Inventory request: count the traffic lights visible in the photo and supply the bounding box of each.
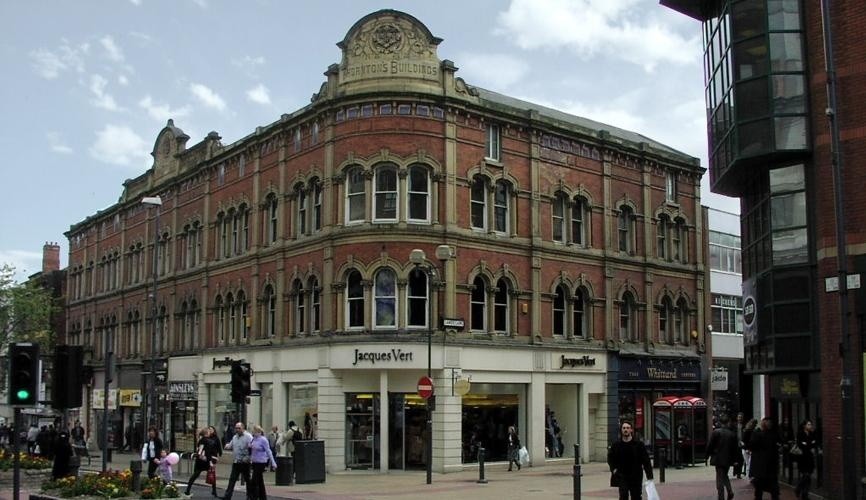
[9,342,39,407]
[230,362,252,400]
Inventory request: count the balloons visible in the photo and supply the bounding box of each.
[166,452,179,465]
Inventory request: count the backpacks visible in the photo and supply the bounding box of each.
[292,428,302,444]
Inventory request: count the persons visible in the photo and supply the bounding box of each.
[607,421,655,500]
[121,420,136,452]
[143,425,163,479]
[222,422,277,499]
[185,426,222,499]
[505,425,522,472]
[267,420,303,472]
[700,411,823,500]
[155,448,172,483]
[1,417,87,480]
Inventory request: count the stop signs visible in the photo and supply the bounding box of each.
[417,376,433,399]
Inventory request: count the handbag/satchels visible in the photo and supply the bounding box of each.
[519,447,529,464]
[643,480,659,500]
[207,467,215,484]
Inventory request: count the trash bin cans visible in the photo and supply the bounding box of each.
[275,456,295,486]
[107,448,118,462]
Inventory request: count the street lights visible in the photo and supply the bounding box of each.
[138,194,162,428]
[408,244,453,486]
